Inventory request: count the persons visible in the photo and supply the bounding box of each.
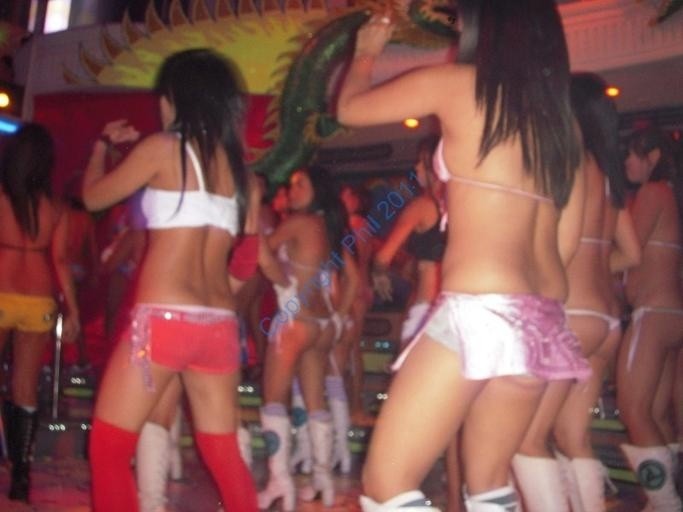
[508,71,642,510]
[0,123,682,510]
[79,49,266,510]
[332,1,584,511]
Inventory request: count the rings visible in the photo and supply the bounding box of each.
[379,16,389,24]
[367,18,375,24]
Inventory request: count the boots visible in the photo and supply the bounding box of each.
[8,403,39,498]
[253,376,351,512]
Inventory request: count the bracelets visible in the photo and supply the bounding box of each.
[92,132,110,147]
